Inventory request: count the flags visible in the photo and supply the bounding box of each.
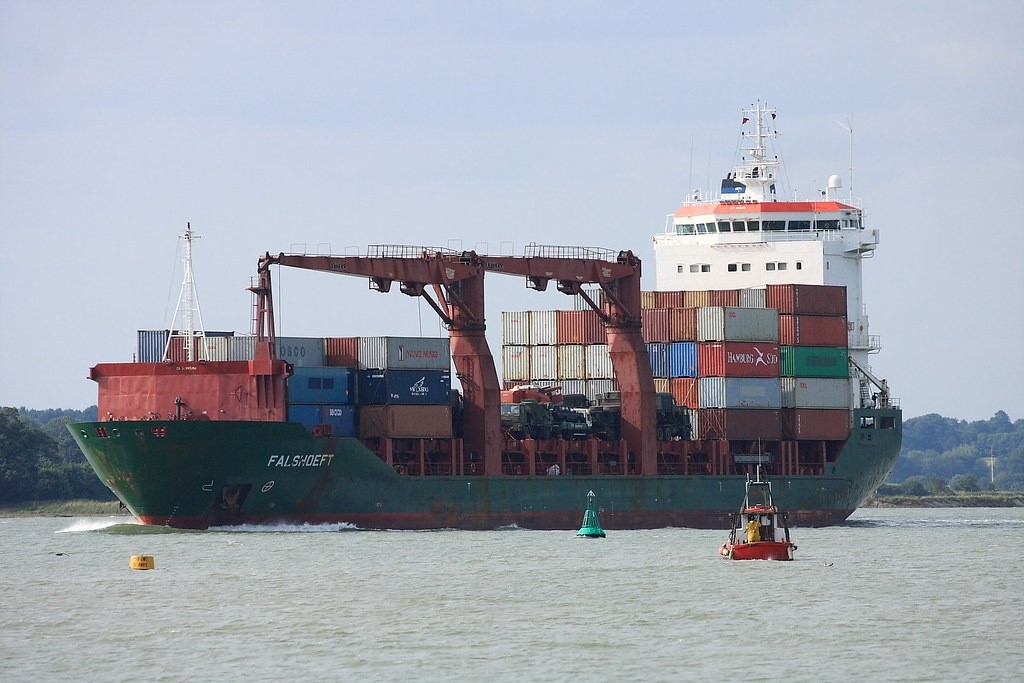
[743,118,749,125]
[772,114,776,120]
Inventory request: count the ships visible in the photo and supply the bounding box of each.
[65,103,904,539]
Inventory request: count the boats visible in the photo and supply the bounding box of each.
[719,467,799,563]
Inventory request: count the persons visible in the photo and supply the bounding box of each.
[872,392,878,409]
[705,459,713,475]
[745,516,762,544]
